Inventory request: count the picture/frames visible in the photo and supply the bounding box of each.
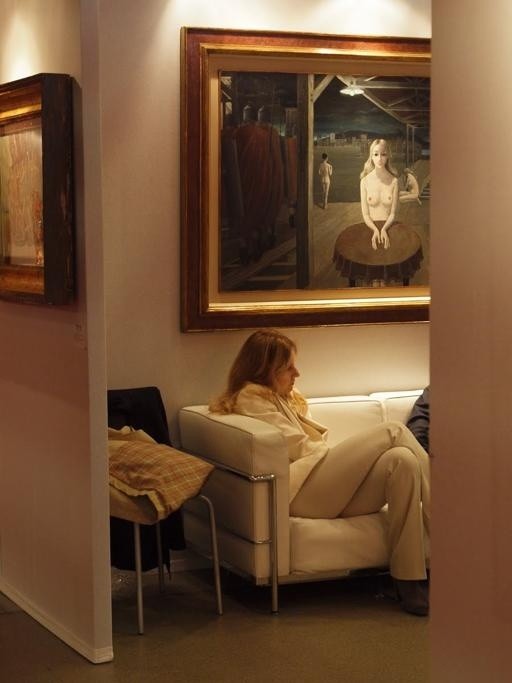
[1,74,74,307]
[181,28,430,334]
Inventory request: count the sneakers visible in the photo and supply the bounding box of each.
[392,578,429,617]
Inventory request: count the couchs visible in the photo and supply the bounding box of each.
[177,389,427,614]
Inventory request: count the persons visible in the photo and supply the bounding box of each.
[398,167,423,206]
[359,137,401,251]
[317,152,333,210]
[405,380,431,455]
[207,327,431,620]
